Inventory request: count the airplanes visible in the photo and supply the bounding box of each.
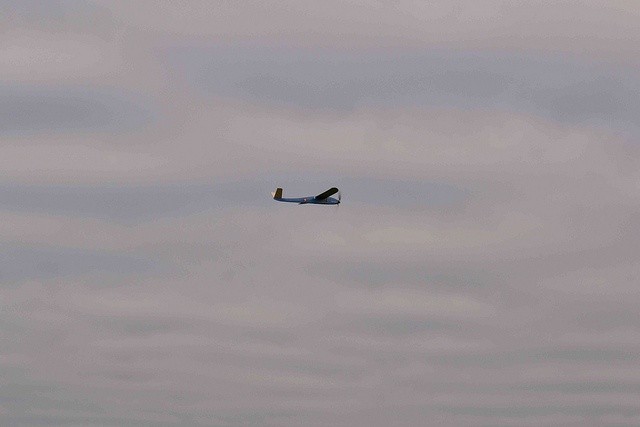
[270,186,343,205]
